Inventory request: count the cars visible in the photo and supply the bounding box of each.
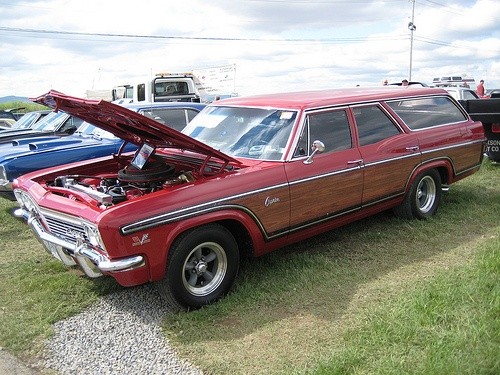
[11,85,487,312]
[427,87,480,102]
[0,103,207,202]
[0,106,85,144]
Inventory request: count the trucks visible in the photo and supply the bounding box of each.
[457,89,500,162]
[132,70,221,103]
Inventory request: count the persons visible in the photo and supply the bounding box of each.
[475,79,490,99]
[382,80,388,87]
[401,79,410,87]
